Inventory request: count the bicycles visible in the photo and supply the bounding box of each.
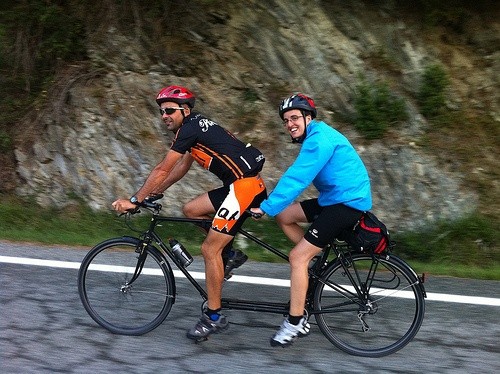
[77,194,428,359]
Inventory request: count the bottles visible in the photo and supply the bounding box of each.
[168,236,193,266]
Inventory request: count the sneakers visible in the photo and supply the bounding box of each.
[309,254,329,275]
[224,249,248,281]
[270,316,311,349]
[185,312,230,343]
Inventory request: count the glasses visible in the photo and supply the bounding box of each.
[159,107,184,115]
[280,114,304,126]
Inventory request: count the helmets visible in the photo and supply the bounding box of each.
[279,94,317,118]
[156,85,196,108]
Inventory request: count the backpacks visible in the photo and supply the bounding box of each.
[345,211,391,255]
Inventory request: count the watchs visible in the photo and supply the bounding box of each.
[130,196,140,206]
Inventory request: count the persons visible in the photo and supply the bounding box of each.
[112,86,268,344]
[250,93,372,347]
